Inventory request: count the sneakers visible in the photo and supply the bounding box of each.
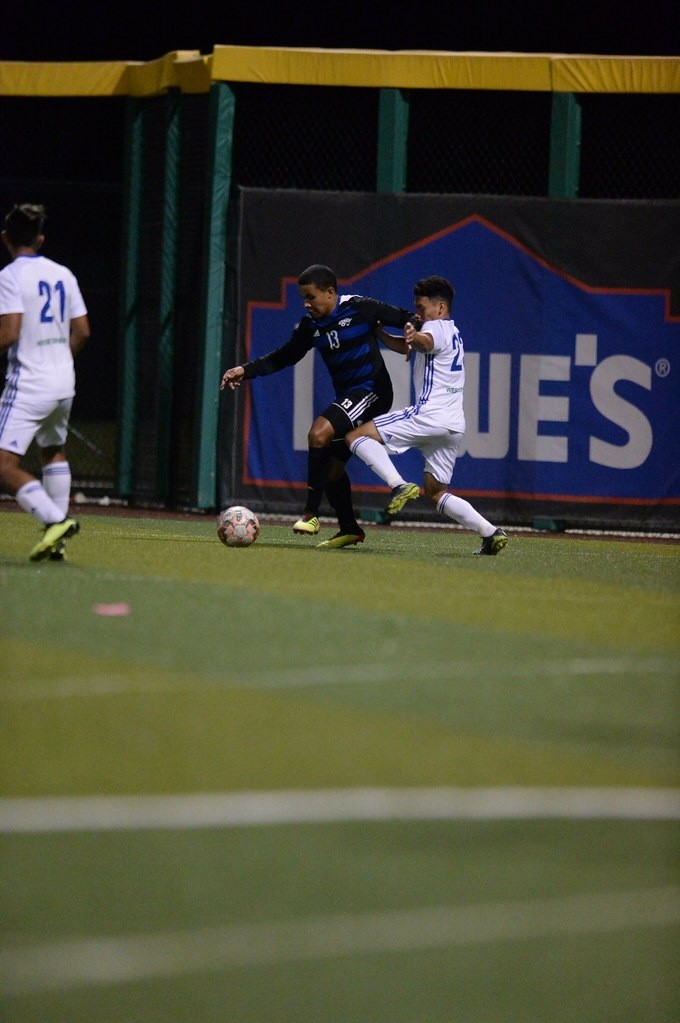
[385,482,420,515]
[28,516,80,561]
[472,527,508,556]
[292,513,320,535]
[50,544,66,561]
[316,525,365,548]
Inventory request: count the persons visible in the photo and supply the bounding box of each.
[219,264,426,550]
[0,202,90,562]
[344,275,510,557]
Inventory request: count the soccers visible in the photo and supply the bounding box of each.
[216,505,261,548]
